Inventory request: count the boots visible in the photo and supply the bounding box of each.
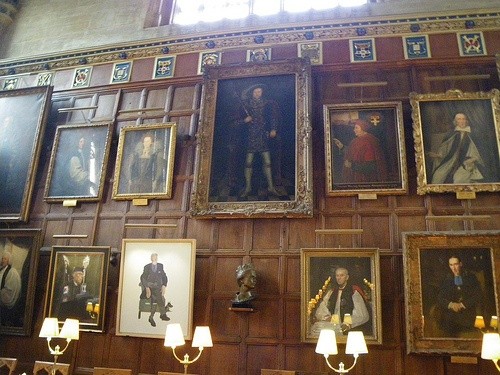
[237,165,252,198]
[262,166,280,194]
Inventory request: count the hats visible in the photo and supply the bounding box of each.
[244,83,267,97]
[354,119,369,131]
[74,267,84,272]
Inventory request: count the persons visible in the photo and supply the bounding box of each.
[229,262,257,307]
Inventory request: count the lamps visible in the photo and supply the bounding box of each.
[163,323,213,375]
[474,315,499,334]
[480,333,500,372]
[330,314,353,333]
[38,318,80,375]
[315,329,369,375]
[85,302,100,320]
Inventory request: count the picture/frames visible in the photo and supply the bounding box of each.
[401,34,432,59]
[0,228,43,337]
[348,38,377,63]
[42,121,115,203]
[115,239,198,341]
[1,77,18,90]
[408,88,500,196]
[456,31,487,57]
[71,66,94,88]
[401,230,500,355]
[246,48,272,62]
[0,84,54,224]
[185,63,316,219]
[196,51,222,75]
[41,245,112,336]
[297,42,324,66]
[322,101,410,198]
[109,61,133,84]
[151,55,177,78]
[111,121,178,200]
[298,246,384,346]
[35,71,56,86]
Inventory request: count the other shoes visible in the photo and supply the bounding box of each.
[149,319,156,327]
[160,315,170,321]
